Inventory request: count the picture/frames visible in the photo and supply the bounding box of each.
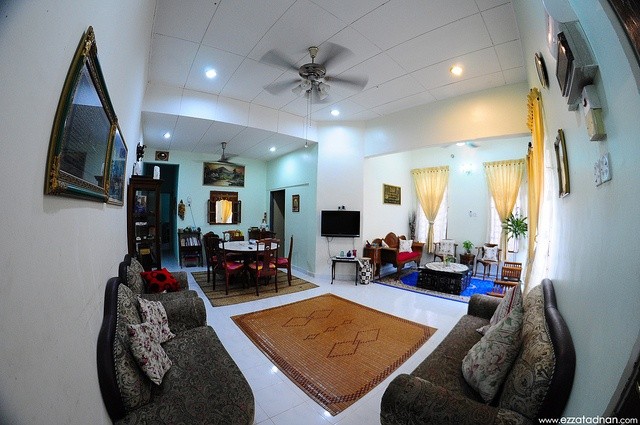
[104,123,129,206]
[44,24,117,202]
[291,195,300,212]
[553,128,570,200]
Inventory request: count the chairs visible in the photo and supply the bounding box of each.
[203,231,221,282]
[434,243,458,263]
[271,234,293,287]
[475,243,502,281]
[248,238,278,297]
[210,237,245,295]
[222,230,241,241]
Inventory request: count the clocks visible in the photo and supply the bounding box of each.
[534,53,547,89]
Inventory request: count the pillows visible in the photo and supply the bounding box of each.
[461,304,525,406]
[125,321,172,385]
[137,296,176,344]
[482,245,498,262]
[381,240,389,248]
[439,239,455,256]
[398,238,413,253]
[475,282,523,335]
[140,267,179,293]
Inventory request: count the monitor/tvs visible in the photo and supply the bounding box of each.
[319,210,361,239]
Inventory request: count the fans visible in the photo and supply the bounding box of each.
[194,142,248,164]
[258,41,367,148]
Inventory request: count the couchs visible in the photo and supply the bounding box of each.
[372,232,425,282]
[118,254,198,302]
[379,278,576,425]
[96,277,255,425]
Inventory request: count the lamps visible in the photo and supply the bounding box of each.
[291,80,333,148]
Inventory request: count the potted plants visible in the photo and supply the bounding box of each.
[462,240,474,255]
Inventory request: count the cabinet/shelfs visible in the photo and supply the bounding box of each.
[129,175,161,274]
[208,191,241,224]
[179,226,203,269]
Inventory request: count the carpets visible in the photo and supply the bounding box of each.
[230,291,438,417]
[370,265,507,305]
[190,266,318,308]
